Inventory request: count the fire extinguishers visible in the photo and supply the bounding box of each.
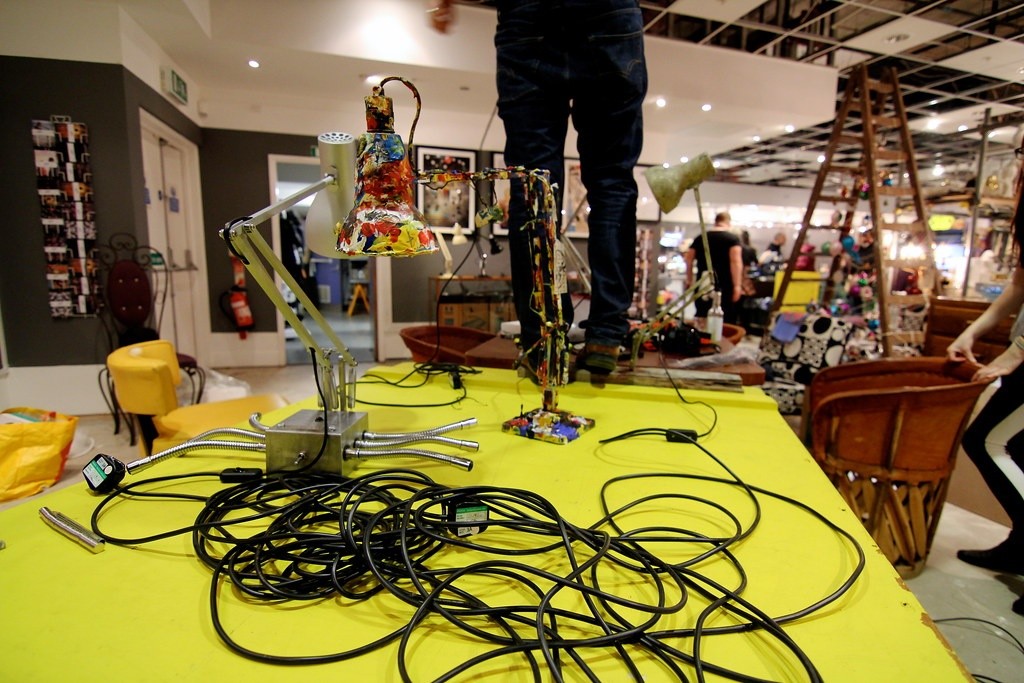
[227,284,255,330]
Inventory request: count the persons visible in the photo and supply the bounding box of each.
[426,0,650,383]
[767,233,786,255]
[683,211,744,327]
[739,231,762,268]
[944,132,1024,616]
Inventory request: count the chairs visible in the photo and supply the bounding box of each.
[86,232,207,448]
[106,341,273,453]
[806,355,993,578]
[398,324,496,367]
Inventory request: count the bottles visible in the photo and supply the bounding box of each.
[706,291,723,341]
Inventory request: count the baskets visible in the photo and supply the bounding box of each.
[0,407,79,502]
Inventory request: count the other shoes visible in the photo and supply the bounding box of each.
[520,352,563,386]
[575,344,620,372]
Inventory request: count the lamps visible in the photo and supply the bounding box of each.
[123,131,481,481]
[434,221,468,278]
[628,150,744,386]
[474,232,504,279]
[335,76,596,445]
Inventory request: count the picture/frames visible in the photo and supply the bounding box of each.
[491,151,510,238]
[632,161,662,225]
[559,154,591,241]
[416,144,480,241]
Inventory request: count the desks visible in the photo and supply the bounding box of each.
[465,332,767,387]
[0,359,975,681]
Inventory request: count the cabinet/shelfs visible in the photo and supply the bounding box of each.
[921,296,1019,366]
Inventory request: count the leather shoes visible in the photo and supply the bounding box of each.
[957,530,1024,575]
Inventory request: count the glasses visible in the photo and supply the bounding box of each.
[1014,147,1024,159]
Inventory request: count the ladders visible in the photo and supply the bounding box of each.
[756,63,944,357]
